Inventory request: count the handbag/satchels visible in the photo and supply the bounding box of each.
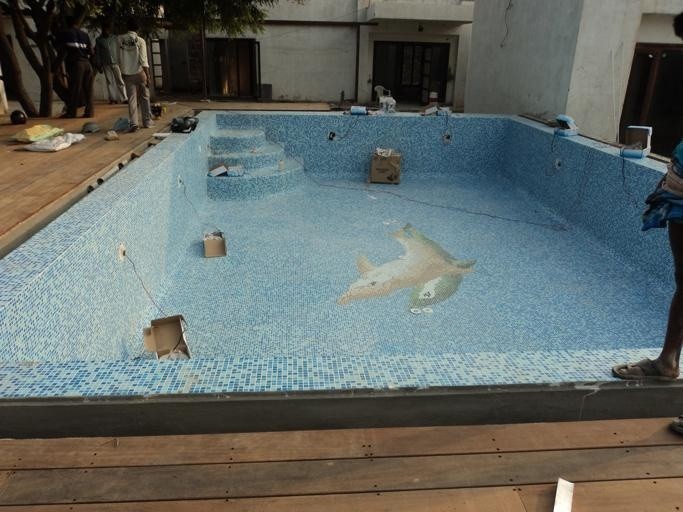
[170,117,199,133]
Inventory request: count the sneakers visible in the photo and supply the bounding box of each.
[83,113,94,117]
[61,113,76,118]
[145,122,157,128]
[130,124,137,132]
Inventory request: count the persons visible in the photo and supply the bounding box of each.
[611,138,682,436]
[53,15,156,131]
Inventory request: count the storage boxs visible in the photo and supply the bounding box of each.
[203,232,227,257]
[143,314,193,361]
[619,125,653,159]
[370,153,401,184]
[553,114,578,137]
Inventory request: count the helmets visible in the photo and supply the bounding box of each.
[11,111,27,124]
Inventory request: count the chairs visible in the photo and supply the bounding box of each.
[374,85,391,108]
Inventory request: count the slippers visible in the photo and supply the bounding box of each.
[612,358,679,381]
[669,416,683,437]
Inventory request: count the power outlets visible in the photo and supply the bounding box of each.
[444,135,451,145]
[177,175,183,188]
[555,159,562,170]
[328,132,335,140]
[118,244,127,262]
